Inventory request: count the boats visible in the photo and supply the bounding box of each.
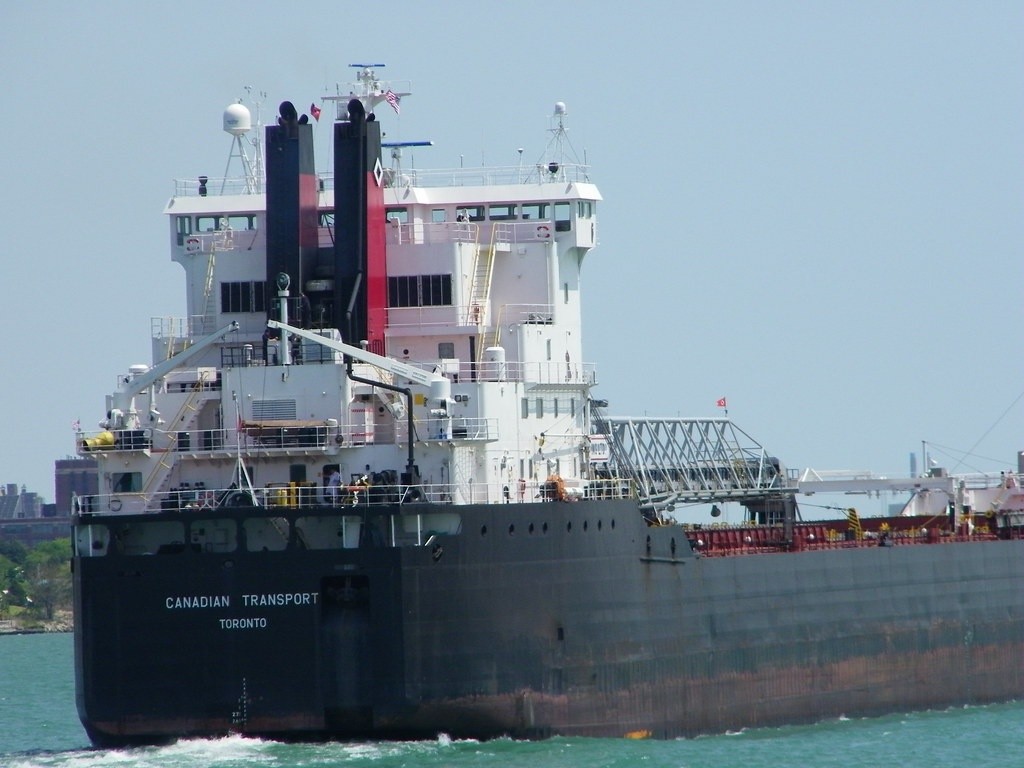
[69,63,1024,746]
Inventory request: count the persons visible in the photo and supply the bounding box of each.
[326,468,343,504]
[286,333,303,367]
[263,330,271,365]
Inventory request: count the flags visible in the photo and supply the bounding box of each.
[717,397,726,406]
[236,414,245,433]
[72,419,80,430]
[311,103,321,122]
[384,90,400,113]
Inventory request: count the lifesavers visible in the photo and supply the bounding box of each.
[199,493,216,508]
[108,500,122,511]
